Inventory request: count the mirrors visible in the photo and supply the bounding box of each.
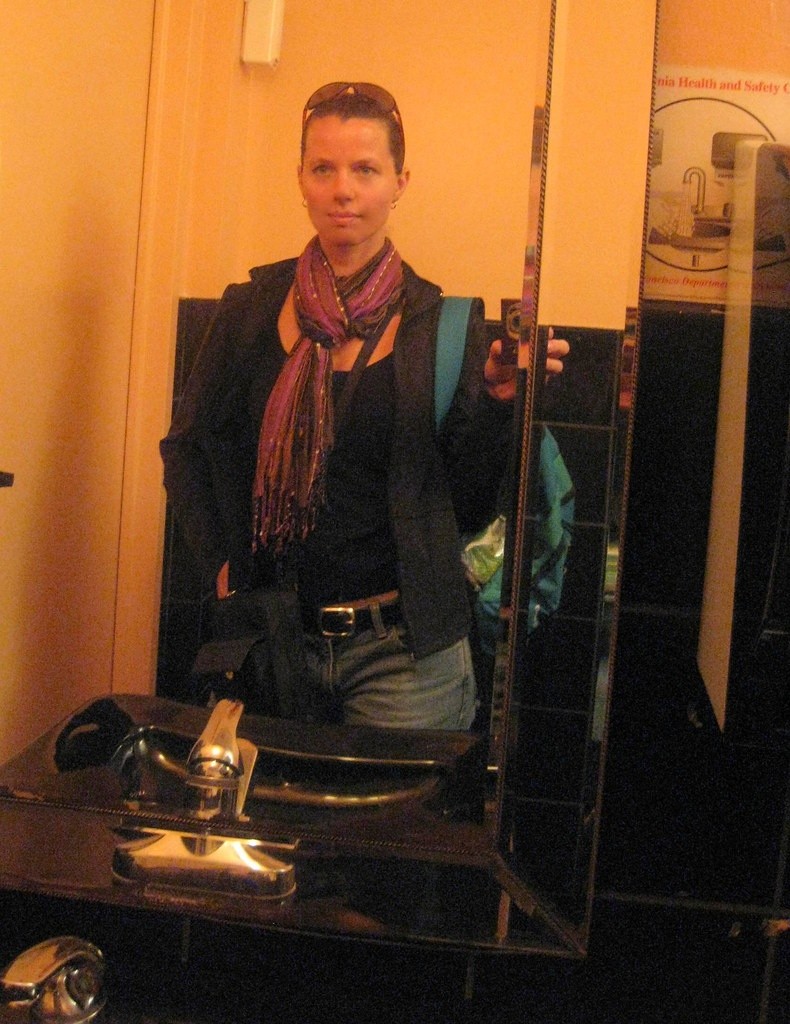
[0,1,661,962]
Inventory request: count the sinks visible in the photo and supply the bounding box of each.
[109,721,449,834]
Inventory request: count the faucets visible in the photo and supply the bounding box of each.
[1,933,119,1023]
[154,697,264,827]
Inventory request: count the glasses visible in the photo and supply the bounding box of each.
[300,82,402,140]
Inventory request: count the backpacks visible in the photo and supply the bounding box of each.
[437,295,578,656]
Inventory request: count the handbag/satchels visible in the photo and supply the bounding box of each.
[191,588,309,718]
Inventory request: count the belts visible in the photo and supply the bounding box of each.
[300,601,402,636]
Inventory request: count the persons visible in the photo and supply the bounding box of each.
[159,81,570,732]
